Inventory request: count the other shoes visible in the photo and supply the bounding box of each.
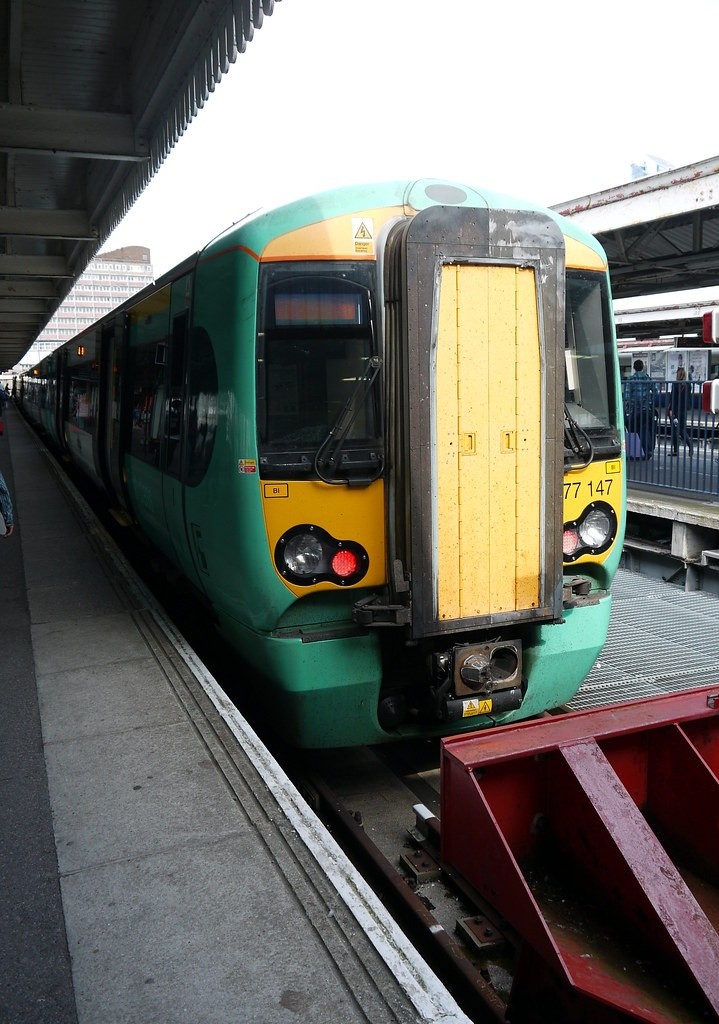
[667,451,678,456]
[643,451,653,460]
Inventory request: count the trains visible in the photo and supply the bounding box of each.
[0,173,628,758]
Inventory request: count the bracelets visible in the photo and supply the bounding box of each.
[10,525,14,528]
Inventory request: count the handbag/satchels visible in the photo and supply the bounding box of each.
[687,390,692,410]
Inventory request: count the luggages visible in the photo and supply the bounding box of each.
[624,425,645,457]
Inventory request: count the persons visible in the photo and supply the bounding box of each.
[678,354,683,367]
[688,364,694,380]
[666,367,694,456]
[623,358,658,461]
[0,472,14,538]
[0,382,11,436]
[69,389,92,430]
